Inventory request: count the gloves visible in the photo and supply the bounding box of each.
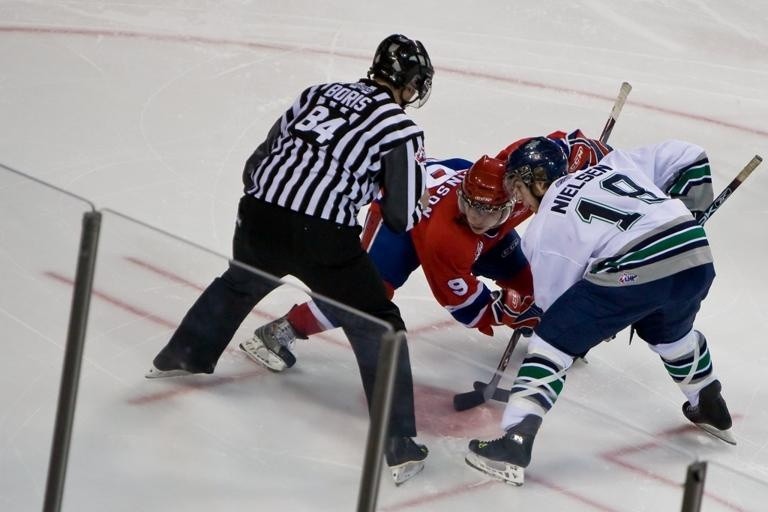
[501,287,542,339]
[570,133,612,171]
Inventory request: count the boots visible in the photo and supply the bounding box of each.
[682,379,732,430]
[255,304,309,367]
[467,413,541,467]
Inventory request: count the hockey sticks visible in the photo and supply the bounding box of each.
[473,156,762,404]
[453,82,632,412]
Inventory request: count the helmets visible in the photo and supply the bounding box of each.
[456,155,516,233]
[503,136,570,207]
[370,34,434,108]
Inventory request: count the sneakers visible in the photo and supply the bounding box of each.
[385,436,428,466]
[153,340,181,371]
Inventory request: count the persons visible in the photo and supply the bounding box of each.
[464,136,735,486]
[143,33,431,486]
[238,131,613,372]
